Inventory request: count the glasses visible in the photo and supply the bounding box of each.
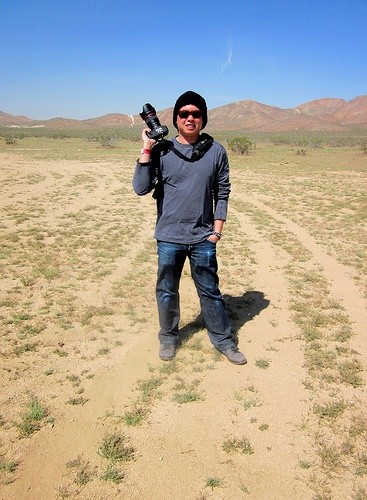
[177,110,202,119]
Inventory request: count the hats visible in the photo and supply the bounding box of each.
[173,91,207,131]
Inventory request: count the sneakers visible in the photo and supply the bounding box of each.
[159,345,175,360]
[221,346,248,365]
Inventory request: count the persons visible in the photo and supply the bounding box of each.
[132,92,247,366]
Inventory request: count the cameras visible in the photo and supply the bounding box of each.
[139,103,169,139]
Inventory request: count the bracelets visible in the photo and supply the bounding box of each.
[212,231,221,239]
[140,148,151,155]
